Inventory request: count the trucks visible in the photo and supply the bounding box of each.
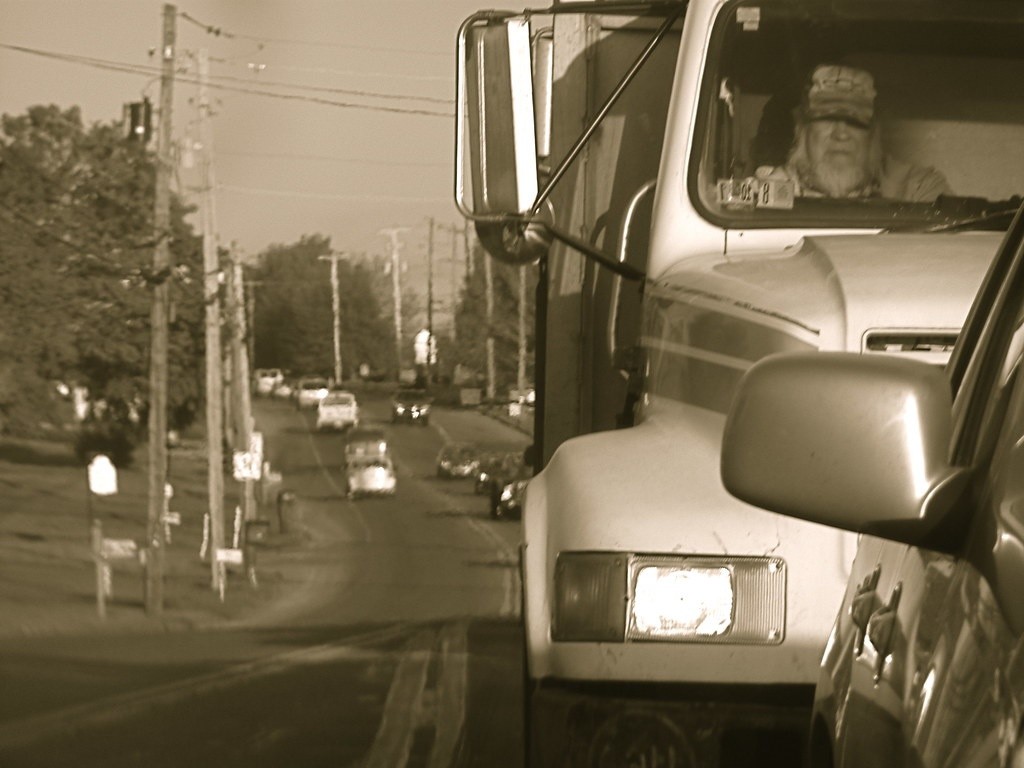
[454,0,1024,766]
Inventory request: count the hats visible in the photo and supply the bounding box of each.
[809,64,876,125]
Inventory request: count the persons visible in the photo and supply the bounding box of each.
[756,64,953,203]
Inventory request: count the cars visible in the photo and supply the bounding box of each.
[718,200,1024,768]
[250,365,331,413]
[439,441,534,520]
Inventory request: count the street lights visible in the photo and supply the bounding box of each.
[317,249,354,388]
[374,225,405,382]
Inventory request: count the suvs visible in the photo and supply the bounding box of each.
[340,424,398,503]
[315,393,362,436]
[388,385,437,428]
